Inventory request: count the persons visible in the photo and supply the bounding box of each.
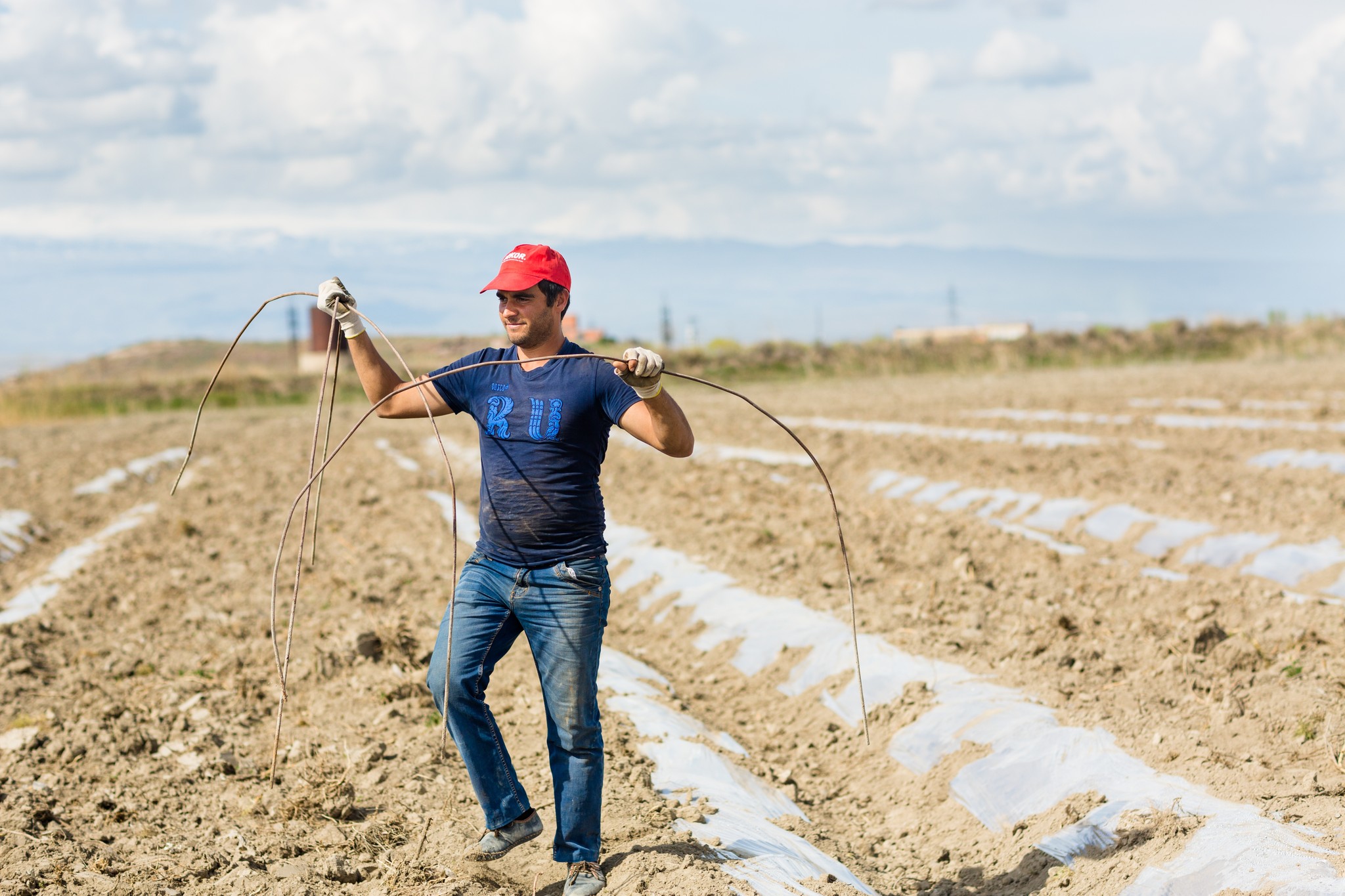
[318,244,695,896]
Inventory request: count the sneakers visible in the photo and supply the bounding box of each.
[463,808,543,861]
[563,862,605,896]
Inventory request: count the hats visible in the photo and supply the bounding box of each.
[480,244,571,293]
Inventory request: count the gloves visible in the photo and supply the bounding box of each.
[614,346,663,398]
[317,277,366,339]
